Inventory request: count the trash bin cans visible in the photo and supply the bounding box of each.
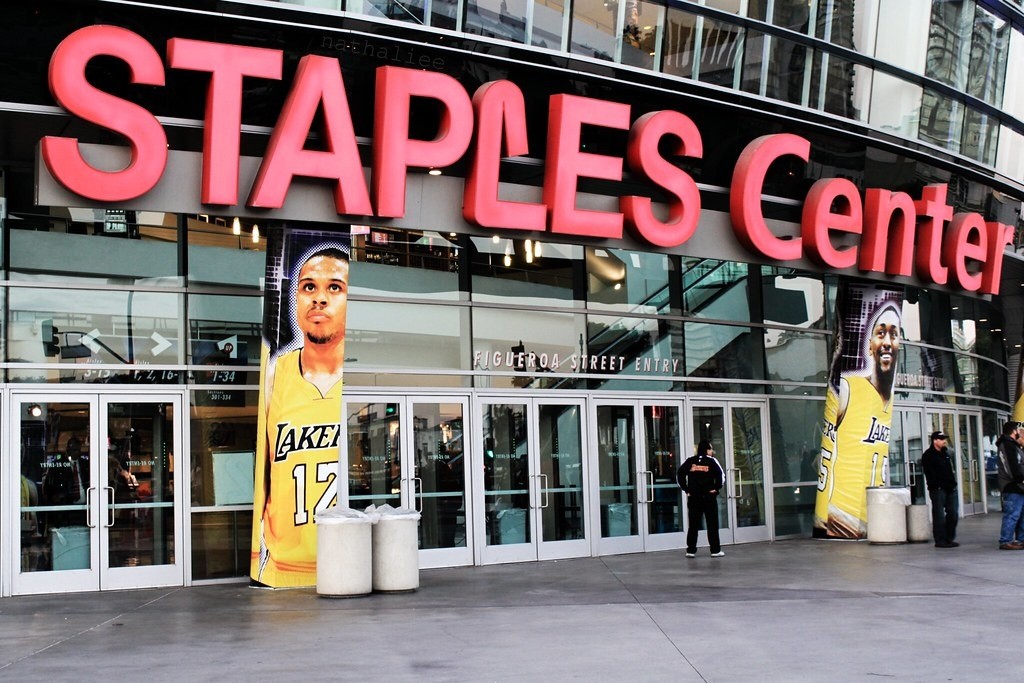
[906,504,930,543]
[865,485,907,545]
[491,508,528,544]
[602,503,634,538]
[372,513,420,594]
[52,527,91,570]
[316,514,372,598]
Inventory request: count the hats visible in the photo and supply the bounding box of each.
[931,431,949,440]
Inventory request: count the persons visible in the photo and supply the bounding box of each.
[252,244,350,589]
[816,305,901,539]
[48,436,90,524]
[677,440,727,558]
[995,421,1024,550]
[921,430,961,549]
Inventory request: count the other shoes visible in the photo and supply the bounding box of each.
[952,542,959,547]
[686,553,695,557]
[935,543,953,548]
[710,550,725,556]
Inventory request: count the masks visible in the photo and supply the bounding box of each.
[1013,429,1020,440]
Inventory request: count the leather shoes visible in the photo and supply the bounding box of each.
[999,544,1024,550]
[1014,542,1024,546]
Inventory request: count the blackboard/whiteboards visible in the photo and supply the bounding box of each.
[211,451,256,506]
